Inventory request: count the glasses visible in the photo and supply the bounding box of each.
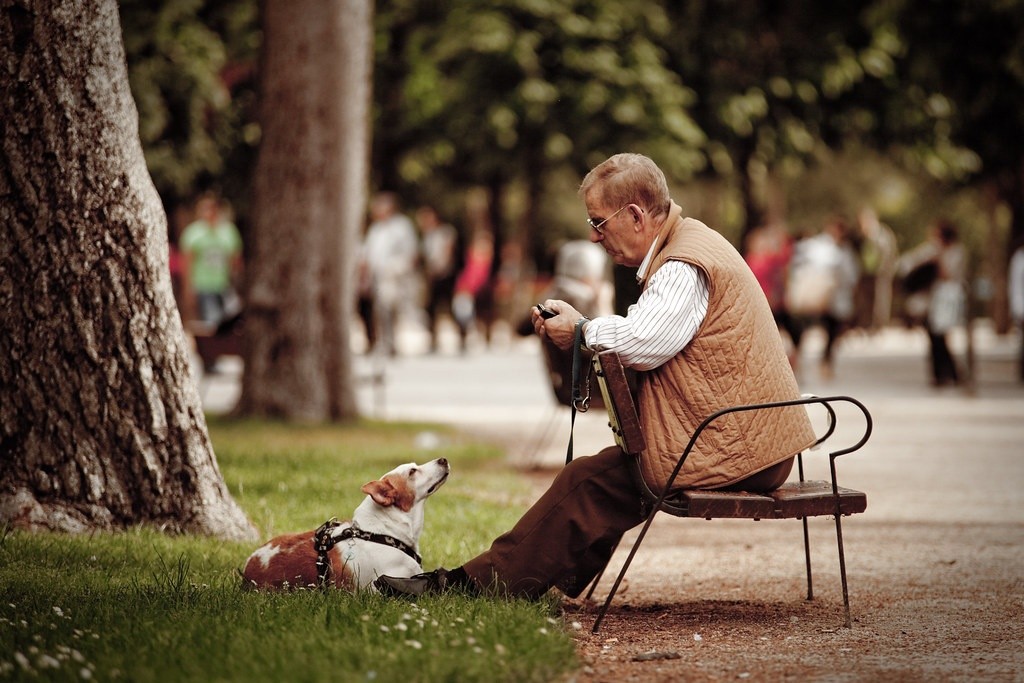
[587,204,644,235]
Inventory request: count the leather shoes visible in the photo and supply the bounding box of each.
[373,568,449,601]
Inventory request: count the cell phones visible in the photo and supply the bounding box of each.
[538,304,558,320]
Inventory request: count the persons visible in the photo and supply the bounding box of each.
[167,189,1024,392]
[374,153,796,603]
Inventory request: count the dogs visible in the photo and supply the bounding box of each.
[238,457,451,594]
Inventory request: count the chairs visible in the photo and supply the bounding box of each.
[584,349,875,634]
